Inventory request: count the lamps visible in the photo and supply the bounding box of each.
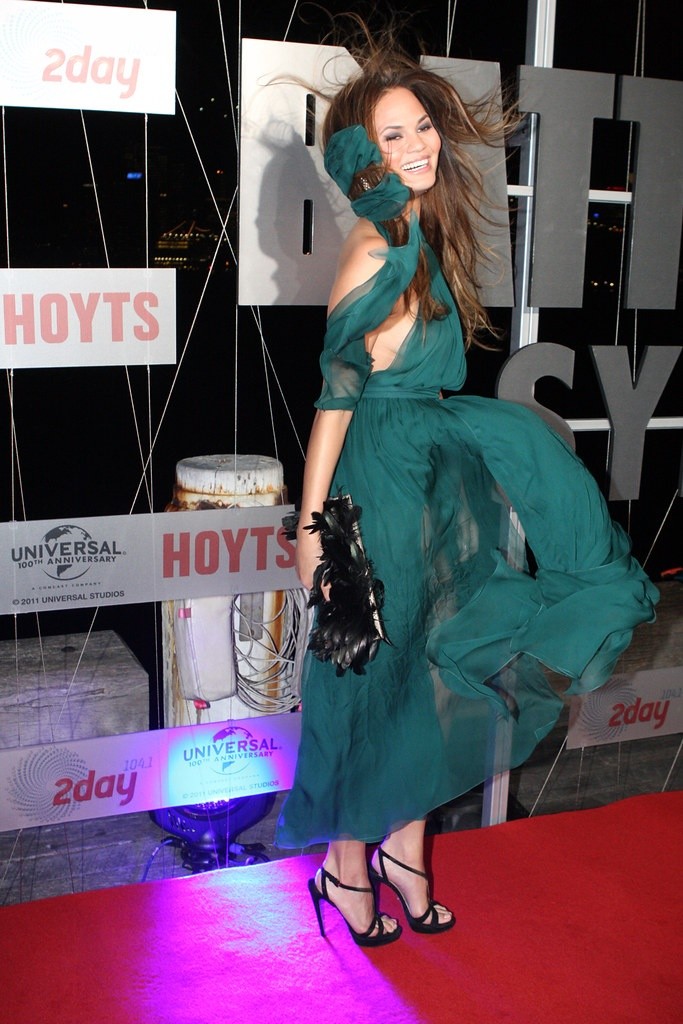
[146,794,275,873]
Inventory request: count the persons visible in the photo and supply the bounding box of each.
[273,62,662,948]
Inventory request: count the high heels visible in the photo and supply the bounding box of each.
[308,866,403,946]
[367,845,457,933]
